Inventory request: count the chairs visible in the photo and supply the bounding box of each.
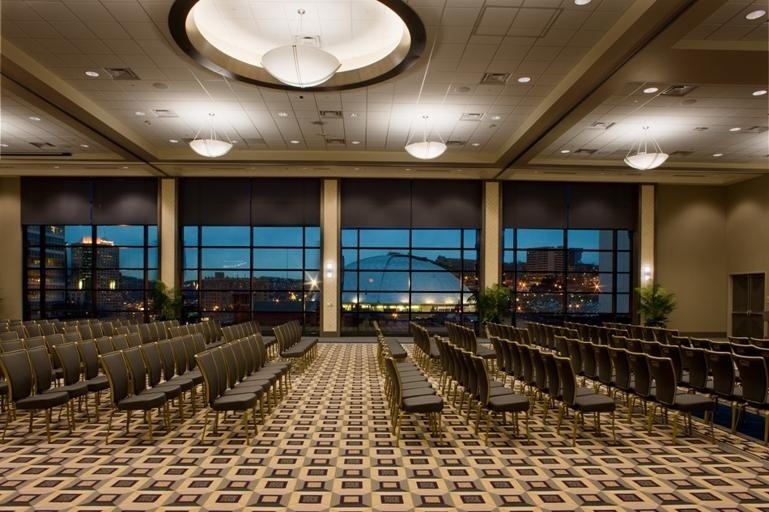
[470,355,531,447]
[646,353,716,446]
[195,351,259,446]
[384,355,445,447]
[2,314,319,430]
[552,354,617,446]
[0,349,71,444]
[98,350,171,444]
[733,352,769,449]
[372,313,768,433]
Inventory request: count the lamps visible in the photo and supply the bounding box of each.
[623,125,672,174]
[403,114,451,162]
[258,10,342,89]
[187,112,236,157]
